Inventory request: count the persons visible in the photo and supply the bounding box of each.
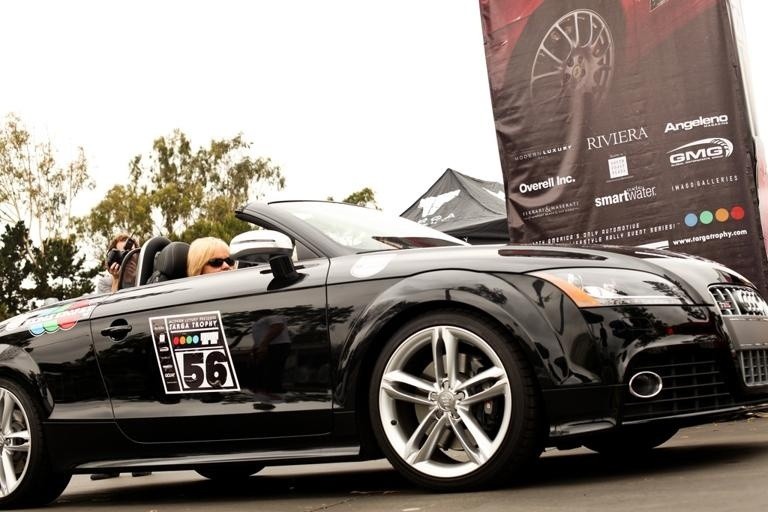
[566,311,675,384]
[183,234,237,279]
[246,304,293,411]
[96,228,143,294]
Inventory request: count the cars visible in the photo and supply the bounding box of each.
[478,1,719,142]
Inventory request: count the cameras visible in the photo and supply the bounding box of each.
[106,237,135,268]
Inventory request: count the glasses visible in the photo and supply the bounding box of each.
[204,258,234,267]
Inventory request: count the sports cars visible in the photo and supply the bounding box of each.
[0,198,767,510]
